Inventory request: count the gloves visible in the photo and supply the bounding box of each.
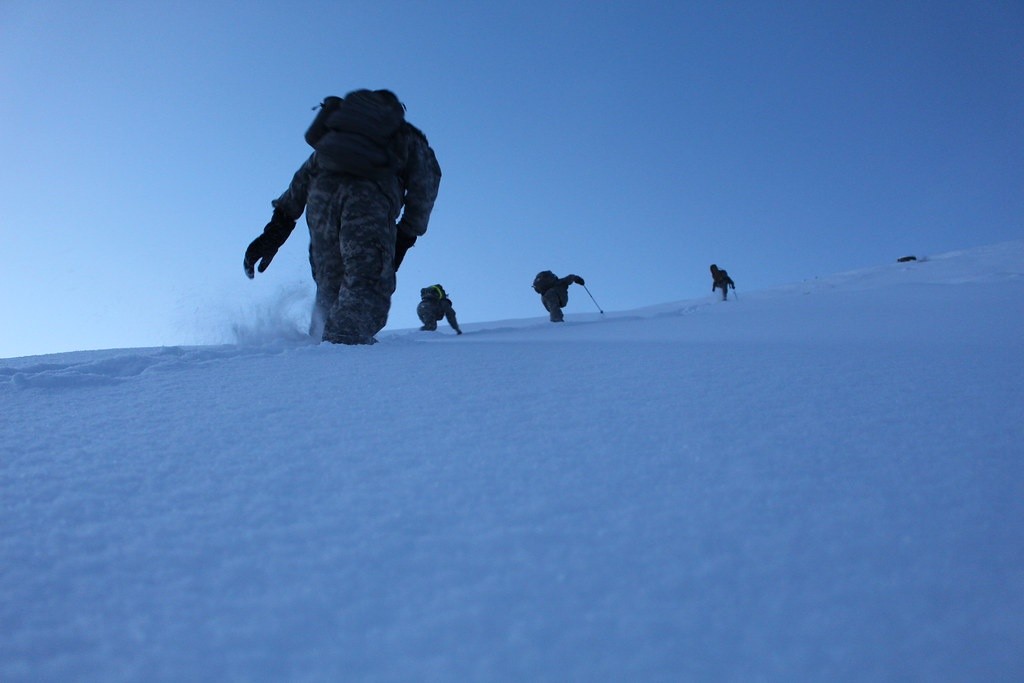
[244,214,296,278]
[392,223,417,272]
[575,276,585,286]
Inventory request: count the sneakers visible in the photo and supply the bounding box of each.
[322,315,379,345]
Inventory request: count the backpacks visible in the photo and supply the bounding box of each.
[313,89,403,176]
[533,269,558,293]
[420,283,447,302]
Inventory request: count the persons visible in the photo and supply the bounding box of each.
[542,275,585,322]
[417,296,463,336]
[710,265,735,301]
[244,89,442,344]
